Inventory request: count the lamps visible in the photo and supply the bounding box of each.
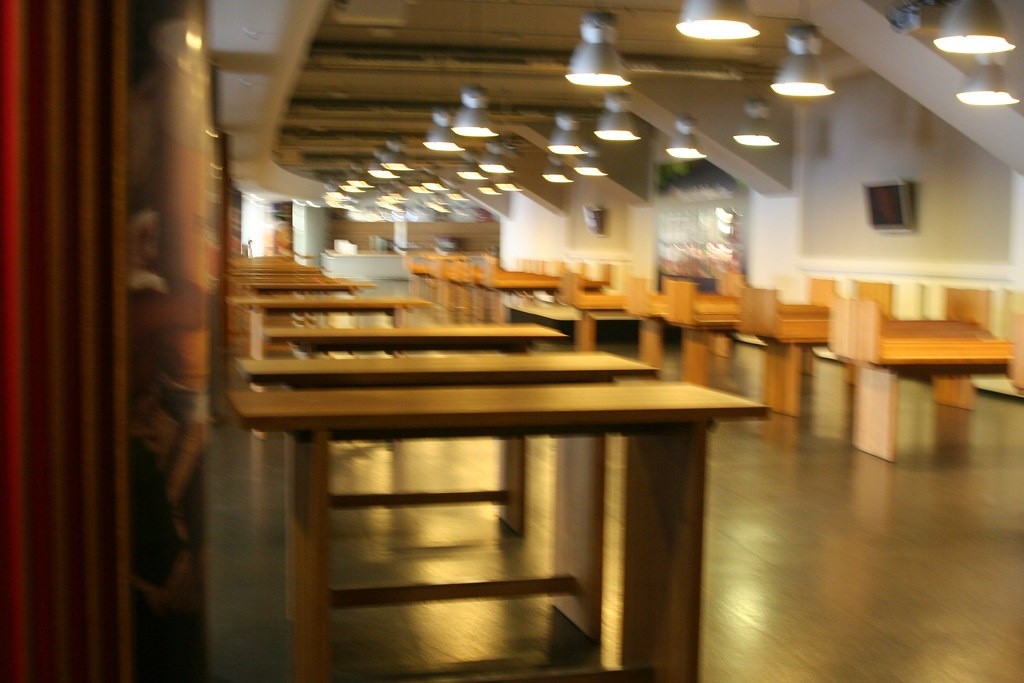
[547,112,590,154]
[541,164,576,183]
[733,96,782,148]
[593,90,643,141]
[565,8,631,87]
[674,0,762,40]
[665,116,707,159]
[932,0,1017,56]
[320,86,524,221]
[573,158,609,176]
[768,0,837,99]
[954,59,1020,106]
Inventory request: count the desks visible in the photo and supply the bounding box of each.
[227,258,1017,683]
